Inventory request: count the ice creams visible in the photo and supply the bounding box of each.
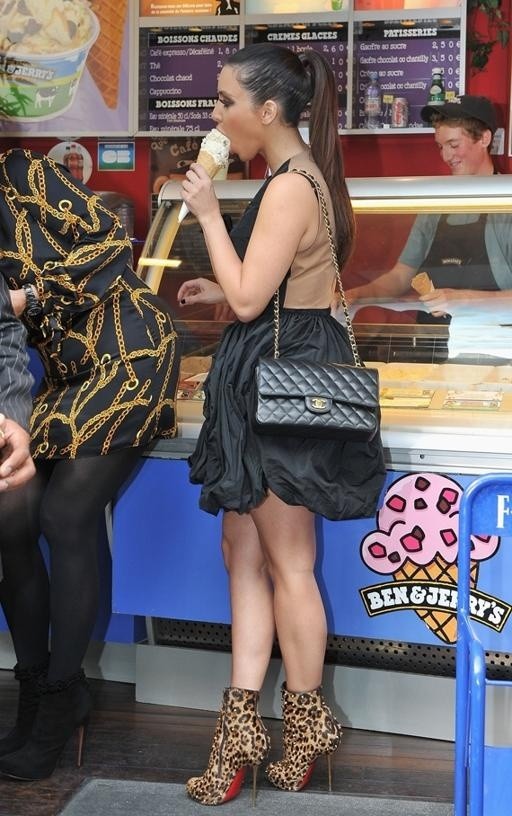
[0,0,127,123]
[178,127,231,222]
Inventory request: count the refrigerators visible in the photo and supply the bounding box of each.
[111,177,512,755]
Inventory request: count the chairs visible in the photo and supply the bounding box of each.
[455,472,512,814]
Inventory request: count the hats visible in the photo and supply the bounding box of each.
[420,95,497,134]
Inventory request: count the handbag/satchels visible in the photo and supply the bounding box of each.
[252,351,381,446]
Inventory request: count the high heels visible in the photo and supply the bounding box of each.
[187,686,270,806]
[265,680,340,794]
[0,660,93,781]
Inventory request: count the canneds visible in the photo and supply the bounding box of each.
[390,98,408,128]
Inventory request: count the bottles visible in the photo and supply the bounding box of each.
[428,67,445,102]
[363,73,380,129]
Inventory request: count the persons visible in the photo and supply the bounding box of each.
[176,43,387,805]
[1,273,37,489]
[335,96,512,325]
[0,147,184,782]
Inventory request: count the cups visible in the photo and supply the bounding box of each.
[1,5,100,124]
